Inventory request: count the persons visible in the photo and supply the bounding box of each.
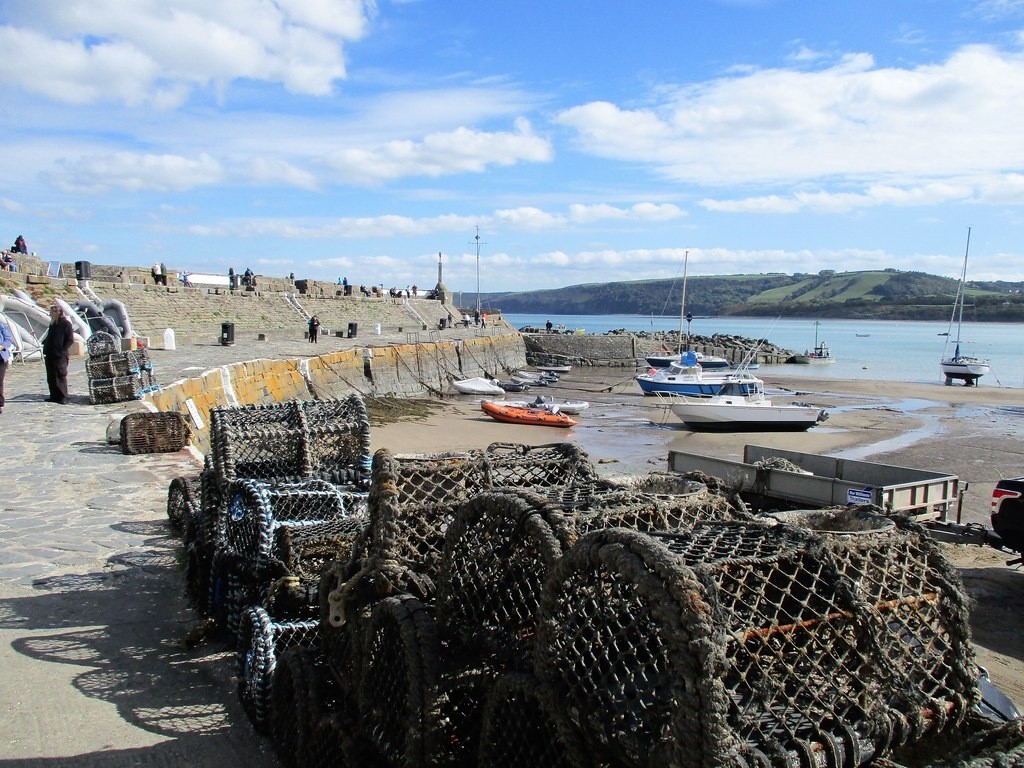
[389,283,418,299]
[474,309,480,325]
[42,306,74,405]
[360,284,372,297]
[0,248,18,272]
[338,277,349,286]
[481,311,487,328]
[178,271,193,288]
[0,323,13,414]
[11,235,27,255]
[153,262,168,286]
[307,316,320,344]
[464,313,470,328]
[447,312,453,329]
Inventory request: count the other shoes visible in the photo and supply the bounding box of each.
[58,399,68,404]
[45,398,56,402]
[0,408,2,414]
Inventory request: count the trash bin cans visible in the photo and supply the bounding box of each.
[440,319,446,329]
[230,276,239,289]
[75,261,91,279]
[222,323,234,346]
[348,323,357,338]
[344,285,352,296]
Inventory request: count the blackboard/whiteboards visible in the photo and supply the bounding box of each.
[47,261,65,277]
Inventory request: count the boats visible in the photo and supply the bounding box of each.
[937,332,951,336]
[855,333,871,337]
[651,378,829,432]
[0,295,132,363]
[452,377,505,395]
[511,375,549,386]
[499,382,525,392]
[478,398,590,414]
[636,315,782,398]
[793,320,836,365]
[481,403,578,426]
[519,371,559,382]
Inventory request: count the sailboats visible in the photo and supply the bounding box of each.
[645,249,730,367]
[939,225,991,388]
